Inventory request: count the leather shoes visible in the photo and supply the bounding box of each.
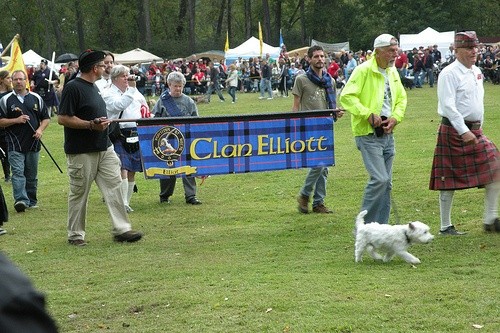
[187,198,202,204]
[160,197,168,202]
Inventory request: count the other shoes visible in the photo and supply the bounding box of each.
[69,239,86,246]
[14,201,26,212]
[483,219,500,231]
[114,230,142,242]
[258,96,266,99]
[5,175,10,182]
[29,200,37,208]
[267,97,273,100]
[438,225,466,235]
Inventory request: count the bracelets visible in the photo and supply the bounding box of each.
[90,120,94,130]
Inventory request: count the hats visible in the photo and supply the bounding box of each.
[41,60,48,65]
[372,34,398,56]
[78,49,107,68]
[454,31,478,47]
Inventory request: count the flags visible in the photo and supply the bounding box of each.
[258,21,263,58]
[0,39,30,93]
[224,31,229,52]
[279,29,284,50]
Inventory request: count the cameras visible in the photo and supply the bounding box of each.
[127,76,140,81]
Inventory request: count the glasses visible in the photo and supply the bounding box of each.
[95,64,107,69]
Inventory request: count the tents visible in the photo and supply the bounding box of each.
[114,48,164,64]
[22,49,59,70]
[400,28,454,49]
[226,36,283,58]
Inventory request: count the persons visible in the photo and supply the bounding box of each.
[231,50,340,94]
[129,57,228,98]
[99,65,146,213]
[224,63,239,103]
[341,44,442,88]
[429,31,500,236]
[276,57,289,98]
[95,50,116,92]
[57,50,142,247]
[445,41,500,85]
[0,70,13,182]
[206,62,226,103]
[27,60,79,117]
[292,45,344,214]
[0,69,51,213]
[338,32,408,238]
[151,70,203,205]
[256,60,274,101]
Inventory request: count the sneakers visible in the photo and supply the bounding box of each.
[299,195,308,213]
[0,228,6,234]
[313,203,333,213]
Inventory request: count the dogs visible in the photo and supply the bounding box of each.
[149,98,156,106]
[271,88,280,98]
[191,93,207,105]
[146,101,150,107]
[354,209,435,265]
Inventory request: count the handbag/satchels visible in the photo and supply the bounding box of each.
[120,129,140,154]
[108,121,120,142]
